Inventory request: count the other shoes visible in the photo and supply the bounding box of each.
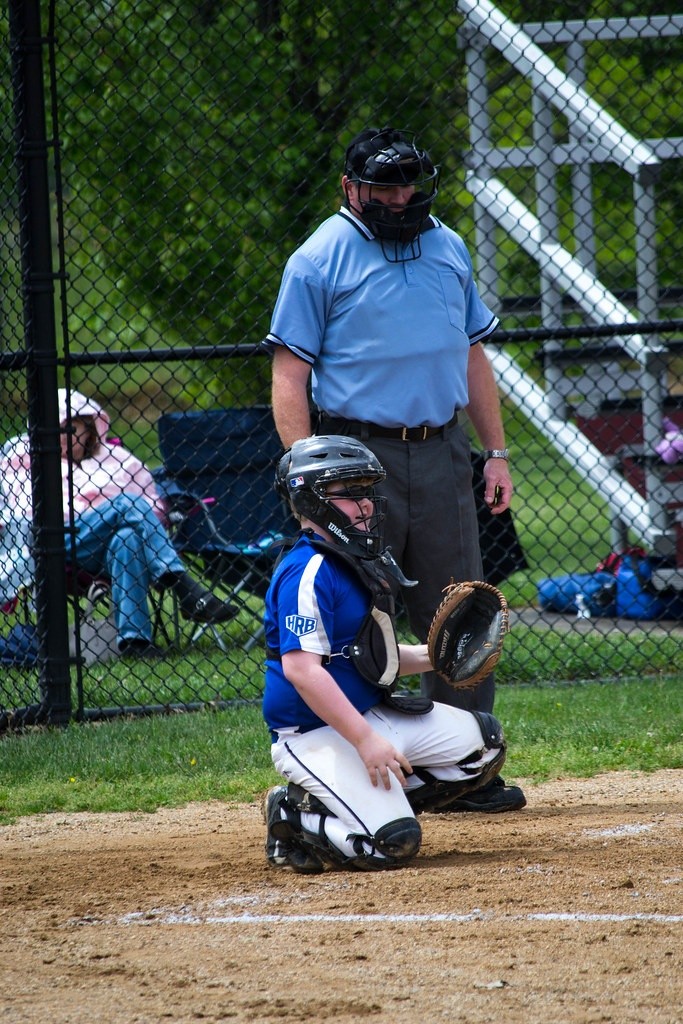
[180,592,240,623]
[125,641,166,658]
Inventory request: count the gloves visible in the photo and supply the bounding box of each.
[273,448,291,501]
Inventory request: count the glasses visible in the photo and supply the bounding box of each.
[325,485,373,501]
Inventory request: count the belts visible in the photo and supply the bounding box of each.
[320,415,458,442]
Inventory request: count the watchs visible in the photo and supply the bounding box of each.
[483,448,509,462]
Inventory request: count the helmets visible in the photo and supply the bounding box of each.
[284,435,387,560]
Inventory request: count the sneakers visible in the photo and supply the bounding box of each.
[265,786,290,865]
[444,783,526,812]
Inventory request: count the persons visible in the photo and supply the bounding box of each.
[263,436,507,874]
[0,387,241,660]
[261,127,527,814]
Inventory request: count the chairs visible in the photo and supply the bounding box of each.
[0,432,177,653]
[150,403,300,651]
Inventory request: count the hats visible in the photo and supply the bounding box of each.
[345,127,434,185]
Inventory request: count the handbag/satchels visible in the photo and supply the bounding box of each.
[68,580,122,663]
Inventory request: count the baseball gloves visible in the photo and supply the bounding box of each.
[424,575,506,689]
